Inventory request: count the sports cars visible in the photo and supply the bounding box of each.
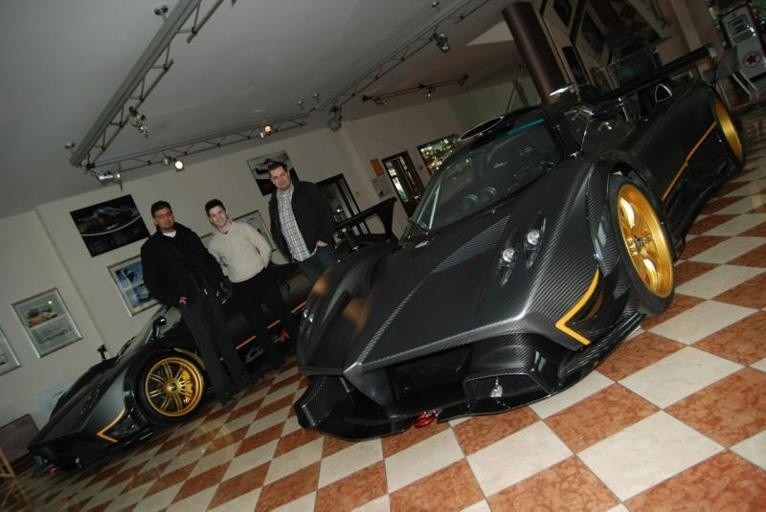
[25,195,399,471]
[293,42,746,444]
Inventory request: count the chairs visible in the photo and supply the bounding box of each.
[709,45,759,111]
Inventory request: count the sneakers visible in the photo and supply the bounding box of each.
[222,377,259,406]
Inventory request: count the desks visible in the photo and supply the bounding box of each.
[325,196,401,258]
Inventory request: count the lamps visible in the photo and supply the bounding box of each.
[95,161,126,187]
[126,107,148,138]
[430,32,451,54]
[368,95,389,110]
[162,155,184,171]
[420,84,437,98]
[262,122,283,137]
[326,104,344,129]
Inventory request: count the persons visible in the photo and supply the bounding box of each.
[206,200,302,373]
[267,159,338,286]
[139,200,260,409]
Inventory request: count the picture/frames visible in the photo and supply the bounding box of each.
[108,208,277,316]
[2,287,83,377]
[589,66,616,91]
